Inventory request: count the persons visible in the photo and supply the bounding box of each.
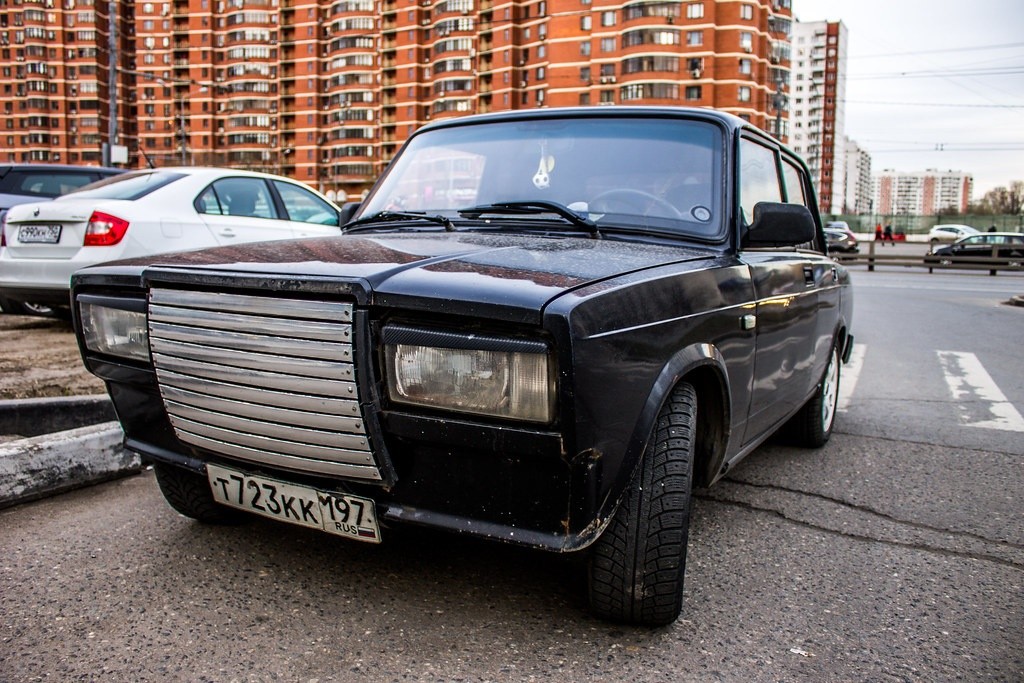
[988,224,997,232]
[875,222,895,247]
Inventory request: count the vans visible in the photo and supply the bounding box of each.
[927,225,983,244]
[0,161,129,243]
[923,232,1024,269]
[824,220,849,230]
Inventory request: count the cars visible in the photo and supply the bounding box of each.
[69,104,854,627]
[822,227,861,264]
[1,166,343,291]
